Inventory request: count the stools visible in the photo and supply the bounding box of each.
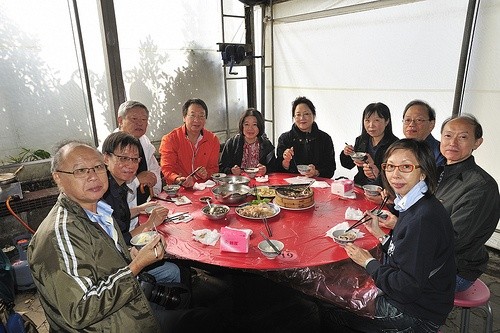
[453,277,495,333]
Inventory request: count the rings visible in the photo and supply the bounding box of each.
[350,252,352,256]
[284,153,286,156]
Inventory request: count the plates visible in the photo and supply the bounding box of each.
[211,173,225,179]
[272,198,316,210]
[249,185,276,198]
[199,196,212,202]
[235,202,280,219]
[243,167,258,173]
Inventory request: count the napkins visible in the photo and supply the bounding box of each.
[283,176,316,185]
[310,179,330,190]
[343,205,365,222]
[169,195,192,206]
[168,211,193,224]
[344,191,358,199]
[188,226,219,247]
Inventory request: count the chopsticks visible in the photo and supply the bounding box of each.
[153,196,176,204]
[262,217,272,238]
[163,212,190,222]
[377,195,391,214]
[256,231,282,255]
[346,206,383,233]
[177,165,205,185]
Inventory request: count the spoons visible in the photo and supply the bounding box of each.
[300,179,316,194]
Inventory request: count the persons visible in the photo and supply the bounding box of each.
[217,108,280,175]
[26,98,221,333]
[275,97,336,180]
[329,100,500,333]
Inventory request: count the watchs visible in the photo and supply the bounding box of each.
[377,234,388,242]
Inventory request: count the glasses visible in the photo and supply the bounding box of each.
[56,163,106,178]
[381,163,421,173]
[402,118,432,125]
[110,152,141,165]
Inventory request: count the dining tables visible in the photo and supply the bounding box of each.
[136,175,393,318]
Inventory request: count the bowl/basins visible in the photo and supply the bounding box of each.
[374,208,391,219]
[332,230,357,245]
[145,206,155,215]
[362,184,382,196]
[350,152,366,161]
[211,185,251,206]
[221,176,251,185]
[258,240,285,260]
[297,165,311,175]
[130,231,158,247]
[201,204,230,220]
[163,185,180,194]
[274,186,315,208]
